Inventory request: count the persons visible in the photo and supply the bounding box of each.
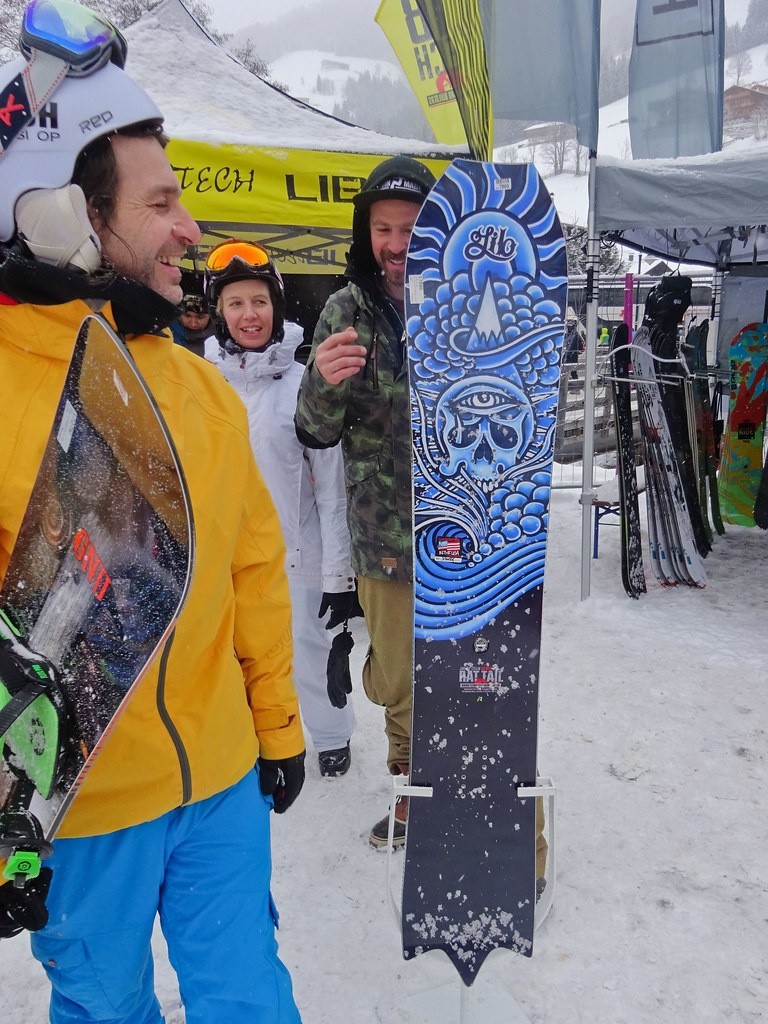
[562,319,581,380]
[292,155,544,903]
[598,327,609,346]
[0,0,311,1024]
[168,288,216,359]
[203,238,361,779]
[580,331,586,340]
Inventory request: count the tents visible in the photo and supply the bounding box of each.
[1,0,475,295]
[579,129,768,598]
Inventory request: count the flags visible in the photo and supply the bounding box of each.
[626,0,725,160]
[477,0,602,151]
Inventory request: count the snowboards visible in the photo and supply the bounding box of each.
[402,156,569,980]
[2,306,197,890]
[611,321,648,601]
[632,318,726,588]
[717,322,768,528]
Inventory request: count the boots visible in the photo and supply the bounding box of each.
[369,763,409,847]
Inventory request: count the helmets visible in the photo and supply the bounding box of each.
[0,58,164,240]
[203,257,285,352]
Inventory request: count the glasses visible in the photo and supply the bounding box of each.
[19,0,127,77]
[205,238,270,274]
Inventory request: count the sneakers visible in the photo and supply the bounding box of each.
[318,739,351,780]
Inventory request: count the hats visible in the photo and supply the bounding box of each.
[179,291,211,316]
[351,176,427,214]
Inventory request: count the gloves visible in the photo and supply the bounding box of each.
[257,747,306,815]
[326,630,353,708]
[318,590,351,630]
[0,867,53,938]
